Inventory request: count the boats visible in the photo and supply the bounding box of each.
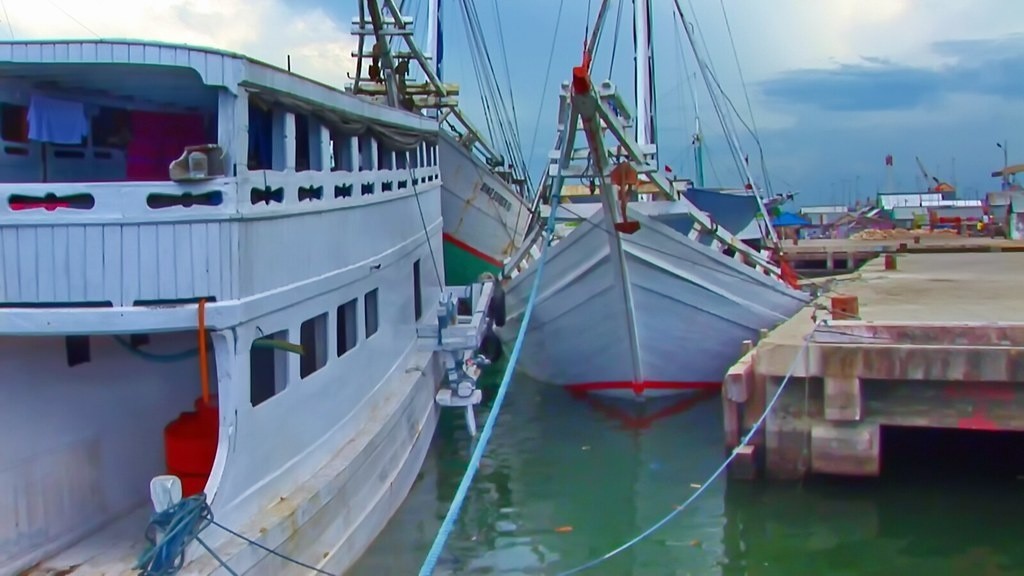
[0,34,511,576]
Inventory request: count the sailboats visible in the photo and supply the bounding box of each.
[491,1,819,433]
[331,0,540,314]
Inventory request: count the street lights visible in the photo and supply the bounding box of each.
[997,142,1007,190]
